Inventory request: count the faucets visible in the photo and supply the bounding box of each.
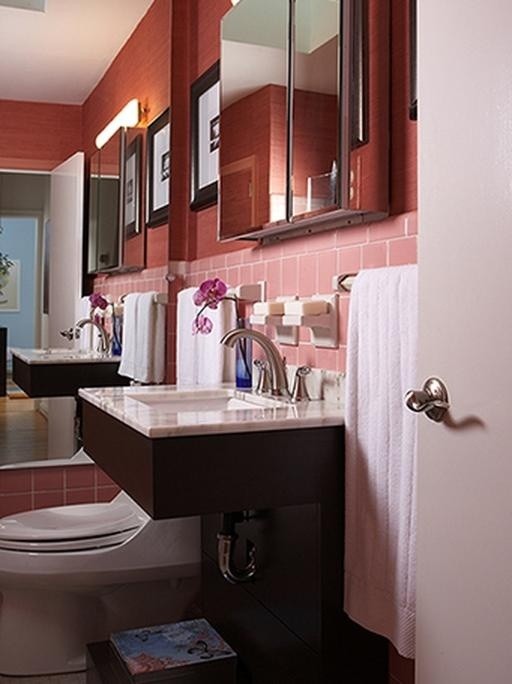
[219,330,291,398]
[74,319,111,352]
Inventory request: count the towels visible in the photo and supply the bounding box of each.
[117,291,168,384]
[176,284,237,385]
[341,266,420,661]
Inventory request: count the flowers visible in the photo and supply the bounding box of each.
[191,278,252,376]
[88,292,122,349]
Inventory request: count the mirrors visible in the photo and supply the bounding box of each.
[0,0,174,471]
[85,126,123,275]
[217,0,344,243]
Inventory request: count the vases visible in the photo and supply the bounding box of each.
[110,315,121,356]
[236,319,253,387]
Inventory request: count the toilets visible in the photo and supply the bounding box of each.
[0,488,202,678]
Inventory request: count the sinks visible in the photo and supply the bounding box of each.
[30,350,98,356]
[126,388,294,413]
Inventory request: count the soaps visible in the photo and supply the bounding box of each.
[284,299,328,316]
[253,301,284,316]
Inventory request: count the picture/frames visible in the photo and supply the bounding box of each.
[145,106,170,227]
[123,134,142,243]
[190,58,229,212]
[0,259,21,313]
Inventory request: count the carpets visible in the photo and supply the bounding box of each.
[6,384,29,399]
